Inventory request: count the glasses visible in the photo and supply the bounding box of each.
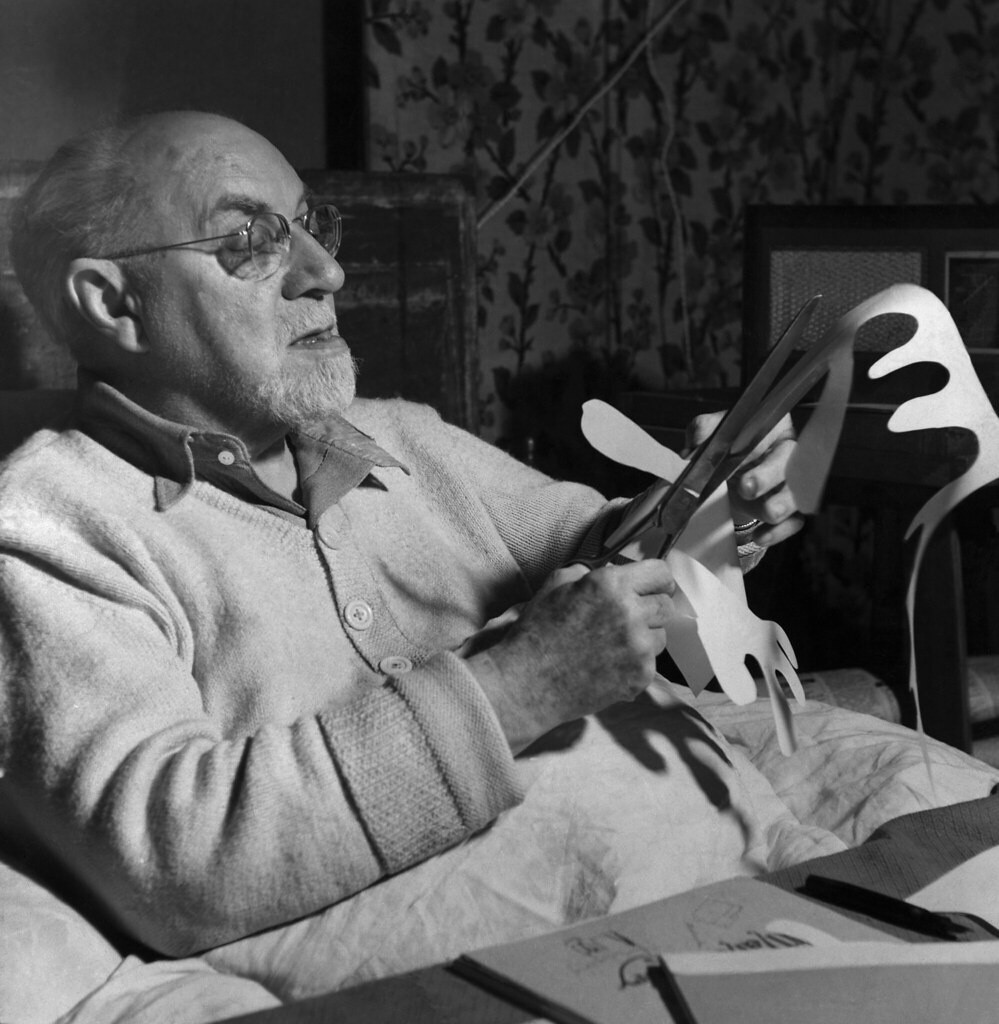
[102,203,343,276]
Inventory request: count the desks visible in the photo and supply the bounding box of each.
[613,387,999,754]
[219,797,999,1024]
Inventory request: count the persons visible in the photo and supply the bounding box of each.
[1,111,806,955]
